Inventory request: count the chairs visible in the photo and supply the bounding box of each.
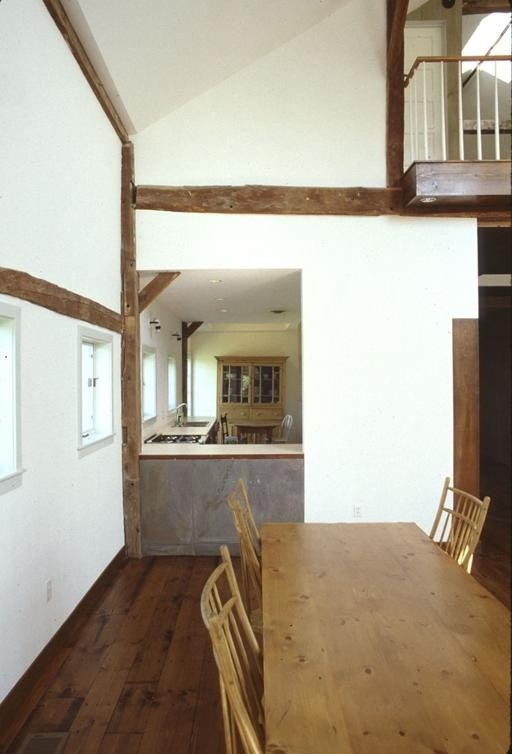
[429,476,491,573]
[264,415,293,444]
[199,546,261,754]
[220,415,247,444]
[225,477,261,635]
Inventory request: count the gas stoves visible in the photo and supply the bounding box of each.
[146,433,203,446]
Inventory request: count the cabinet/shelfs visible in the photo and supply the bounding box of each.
[214,355,289,444]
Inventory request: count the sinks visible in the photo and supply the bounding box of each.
[170,420,212,428]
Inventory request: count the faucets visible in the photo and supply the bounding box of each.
[176,403,187,423]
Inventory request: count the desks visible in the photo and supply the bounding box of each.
[235,423,276,443]
[261,522,511,754]
